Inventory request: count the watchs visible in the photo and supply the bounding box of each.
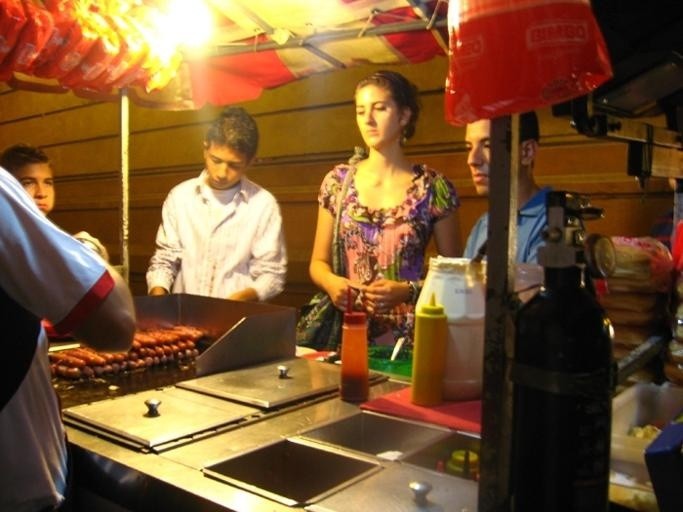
[76,237,101,262]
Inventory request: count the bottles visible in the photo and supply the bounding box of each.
[340,287,449,406]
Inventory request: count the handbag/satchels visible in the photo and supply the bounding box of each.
[294,291,342,351]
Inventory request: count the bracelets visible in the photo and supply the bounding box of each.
[407,279,420,309]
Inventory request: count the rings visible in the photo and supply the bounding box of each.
[380,302,386,311]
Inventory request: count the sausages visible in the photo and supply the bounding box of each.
[49,325,204,379]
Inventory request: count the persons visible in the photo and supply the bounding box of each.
[0,164,136,510]
[296,71,462,351]
[147,107,288,304]
[0,144,76,343]
[462,110,554,378]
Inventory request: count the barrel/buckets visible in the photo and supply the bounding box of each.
[415,255,486,401]
